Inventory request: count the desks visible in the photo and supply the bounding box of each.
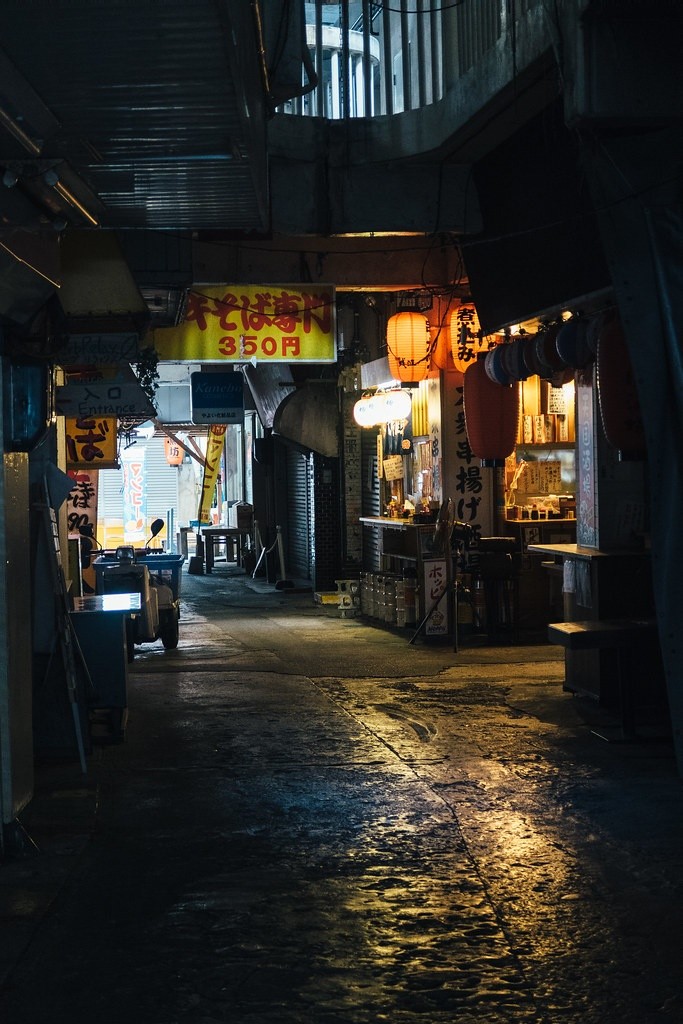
[202,528,250,574]
[71,591,144,741]
[361,515,455,644]
[524,542,652,562]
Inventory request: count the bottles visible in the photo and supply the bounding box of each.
[548,508,553,519]
[391,504,397,517]
[532,504,538,519]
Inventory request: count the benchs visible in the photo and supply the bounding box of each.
[548,616,672,743]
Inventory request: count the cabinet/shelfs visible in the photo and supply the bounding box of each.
[495,441,578,628]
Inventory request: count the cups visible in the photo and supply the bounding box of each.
[522,510,529,520]
[568,511,573,519]
[540,509,545,519]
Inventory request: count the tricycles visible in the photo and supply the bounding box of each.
[77,518,186,665]
